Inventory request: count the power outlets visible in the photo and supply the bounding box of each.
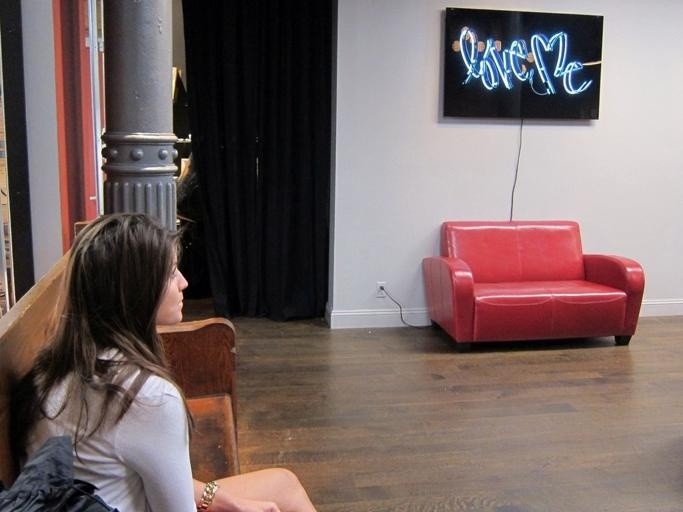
[375,281,387,300]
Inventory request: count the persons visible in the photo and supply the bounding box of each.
[5,212,319,511]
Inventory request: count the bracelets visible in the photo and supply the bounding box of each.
[195,480,219,511]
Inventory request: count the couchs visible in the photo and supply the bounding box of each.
[421,220,645,353]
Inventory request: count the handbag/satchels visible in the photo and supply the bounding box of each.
[0,436,120,512]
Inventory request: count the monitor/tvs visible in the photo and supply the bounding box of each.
[444,7,604,120]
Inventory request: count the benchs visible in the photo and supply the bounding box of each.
[0,241,241,512]
